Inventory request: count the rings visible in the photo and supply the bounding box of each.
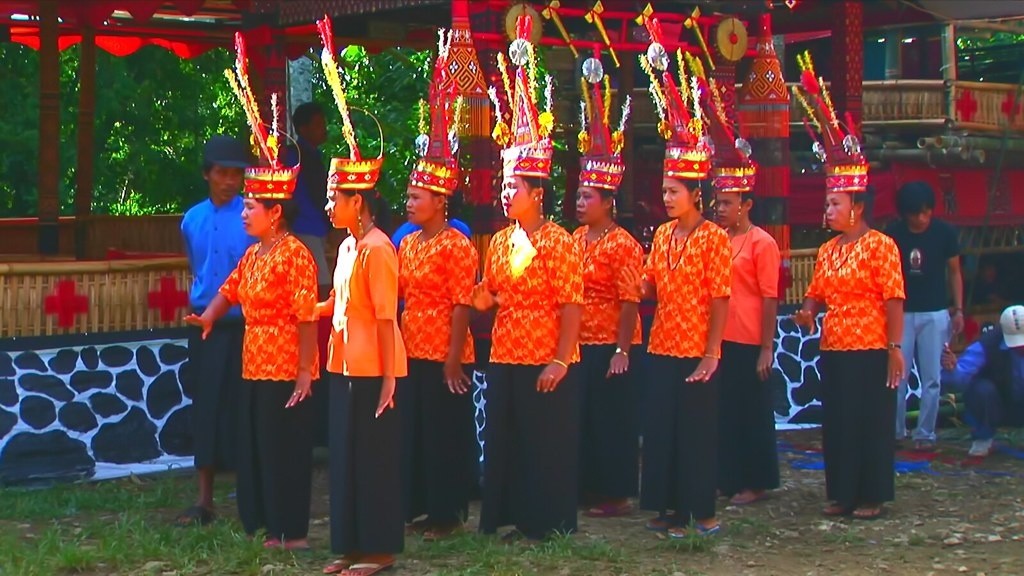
[457,379,462,383]
[293,392,297,397]
[701,370,706,374]
[549,375,554,379]
[297,390,302,394]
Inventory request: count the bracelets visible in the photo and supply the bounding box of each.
[704,354,720,358]
[553,359,568,368]
[955,308,963,312]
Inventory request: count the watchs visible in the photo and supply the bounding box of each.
[616,347,629,356]
[888,343,901,349]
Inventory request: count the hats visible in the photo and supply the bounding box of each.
[202,134,248,176]
[999,305,1024,348]
[224,0,872,199]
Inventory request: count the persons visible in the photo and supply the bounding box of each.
[939,305,1024,457]
[882,180,964,452]
[287,101,338,302]
[787,182,905,518]
[639,177,733,541]
[715,188,781,506]
[471,175,583,541]
[173,133,258,530]
[571,183,643,517]
[180,186,480,576]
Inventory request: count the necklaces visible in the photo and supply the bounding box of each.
[406,225,445,270]
[247,230,290,282]
[355,222,374,239]
[724,224,752,261]
[830,230,864,270]
[667,220,703,271]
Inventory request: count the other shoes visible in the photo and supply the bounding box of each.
[500,529,544,545]
[263,538,310,551]
[916,440,935,451]
[969,438,994,458]
[896,440,905,448]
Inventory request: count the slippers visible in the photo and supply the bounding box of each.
[423,530,449,540]
[731,493,770,505]
[323,558,351,574]
[338,561,396,576]
[583,504,632,518]
[646,515,684,529]
[668,521,720,539]
[176,506,210,526]
[821,503,889,519]
